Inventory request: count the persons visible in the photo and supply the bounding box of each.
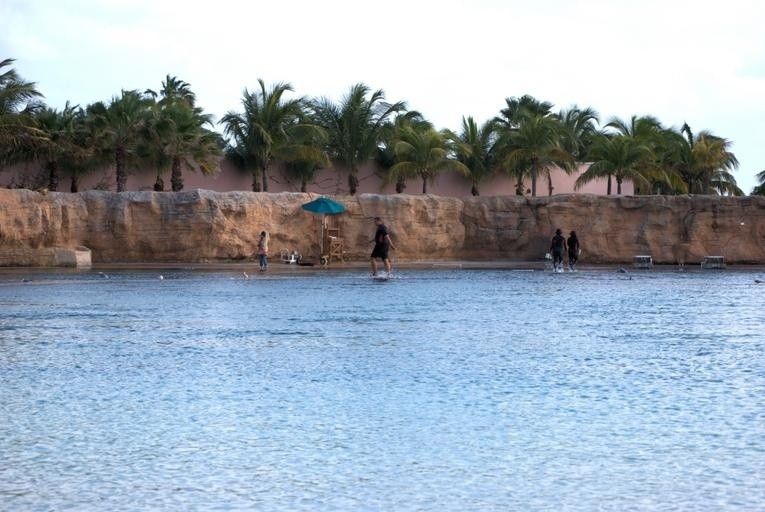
[549,228,568,273]
[367,216,398,277]
[566,230,580,272]
[255,230,268,273]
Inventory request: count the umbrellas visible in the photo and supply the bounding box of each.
[301,196,348,264]
